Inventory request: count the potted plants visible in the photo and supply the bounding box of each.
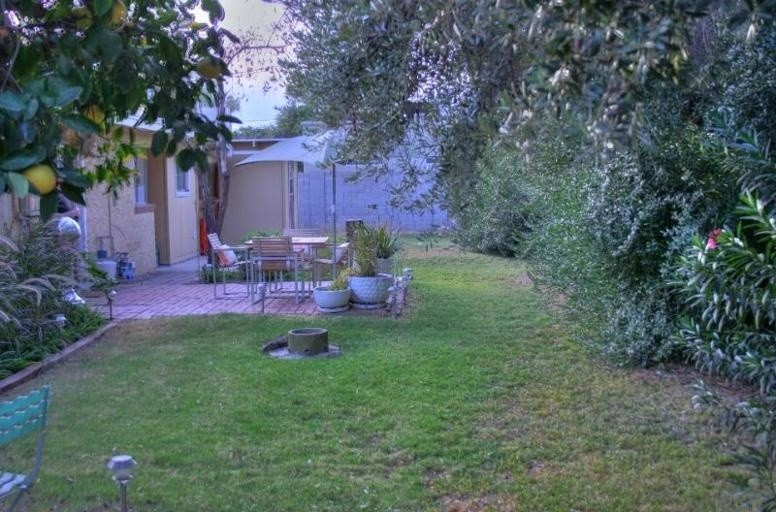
[310,216,404,312]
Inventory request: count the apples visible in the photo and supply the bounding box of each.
[84,106,106,124]
[109,0,126,25]
[72,6,93,30]
[21,164,57,194]
[199,56,222,77]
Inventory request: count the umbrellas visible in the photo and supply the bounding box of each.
[229,130,437,284]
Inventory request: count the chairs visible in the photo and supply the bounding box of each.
[0,384,50,512]
[206,227,351,314]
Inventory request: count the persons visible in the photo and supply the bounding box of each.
[50,178,84,305]
